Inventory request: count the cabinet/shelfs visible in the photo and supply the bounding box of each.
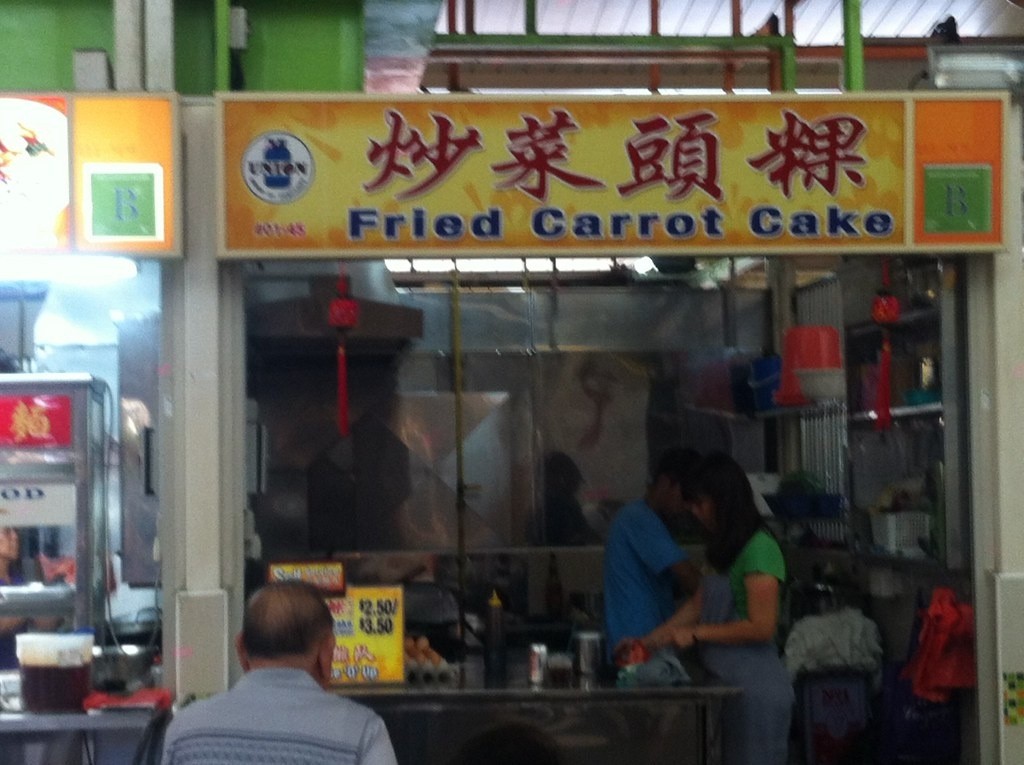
[838,259,971,569]
[0,374,110,632]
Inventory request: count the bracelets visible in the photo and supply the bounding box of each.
[691,632,701,642]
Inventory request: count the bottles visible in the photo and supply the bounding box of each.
[575,633,608,690]
[529,644,546,690]
[484,591,505,688]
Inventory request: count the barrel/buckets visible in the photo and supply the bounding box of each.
[14,630,94,713]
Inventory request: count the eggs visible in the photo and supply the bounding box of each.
[403,634,441,667]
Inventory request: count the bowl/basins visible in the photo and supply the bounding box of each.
[94,645,159,693]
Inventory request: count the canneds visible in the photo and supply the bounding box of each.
[529,643,548,684]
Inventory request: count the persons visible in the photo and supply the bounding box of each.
[0,527,64,670]
[602,446,796,765]
[161,578,398,765]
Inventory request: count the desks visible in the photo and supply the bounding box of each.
[325,668,746,765]
[1,688,164,765]
